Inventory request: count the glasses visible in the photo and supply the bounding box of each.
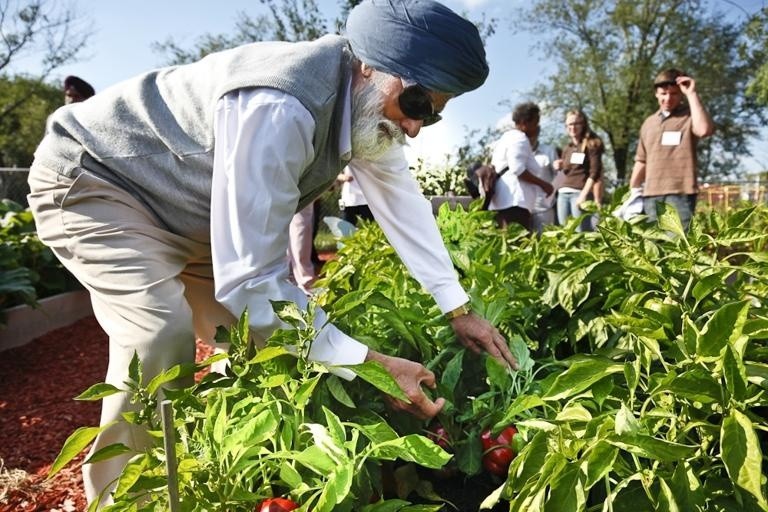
[397,74,442,127]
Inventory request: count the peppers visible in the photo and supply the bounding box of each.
[428,428,448,447]
[480,426,518,475]
[255,497,295,511]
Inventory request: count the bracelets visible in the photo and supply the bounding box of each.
[443,300,476,320]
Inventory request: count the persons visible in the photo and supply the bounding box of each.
[332,164,373,226]
[486,100,608,238]
[25,0,526,511]
[627,66,716,240]
[64,75,95,106]
[286,196,323,290]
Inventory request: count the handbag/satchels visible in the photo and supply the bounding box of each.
[464,164,497,211]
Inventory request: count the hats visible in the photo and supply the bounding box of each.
[653,66,688,85]
